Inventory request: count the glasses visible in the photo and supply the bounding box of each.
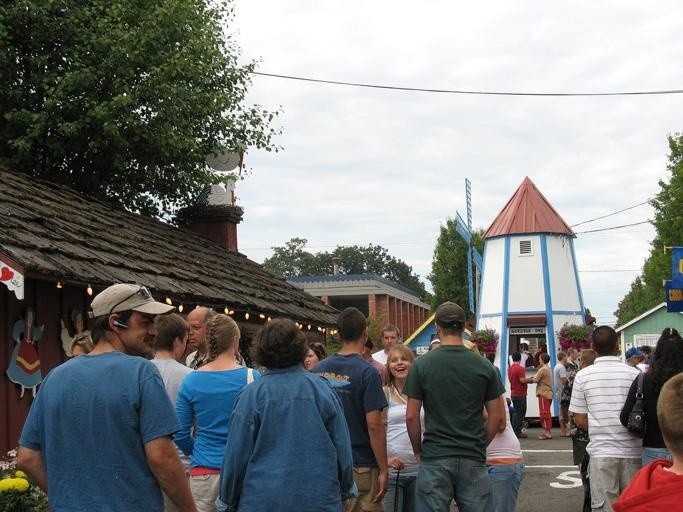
[309,342,324,352]
[111,285,152,313]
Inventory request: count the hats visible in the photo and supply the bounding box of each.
[626,346,643,358]
[435,301,466,329]
[88,284,176,319]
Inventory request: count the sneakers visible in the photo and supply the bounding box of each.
[518,433,527,439]
[538,433,552,440]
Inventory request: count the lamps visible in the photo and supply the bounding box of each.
[165,296,172,305]
[224,306,228,314]
[86,282,94,296]
[179,302,184,312]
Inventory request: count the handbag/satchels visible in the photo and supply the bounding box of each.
[627,399,647,434]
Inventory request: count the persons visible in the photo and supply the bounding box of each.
[146,299,525,511]
[70,333,96,358]
[559,324,682,511]
[16,282,198,512]
[509,342,567,439]
